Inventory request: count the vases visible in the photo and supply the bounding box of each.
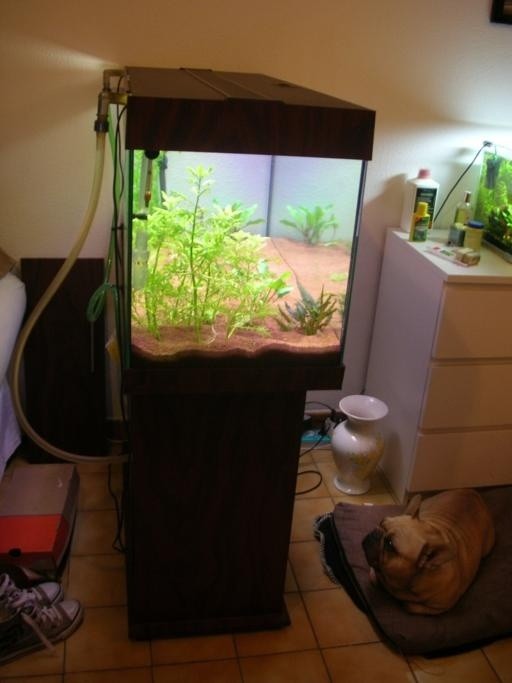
[330,394,390,496]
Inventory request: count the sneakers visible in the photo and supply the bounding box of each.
[0,572,65,620]
[1,598,82,665]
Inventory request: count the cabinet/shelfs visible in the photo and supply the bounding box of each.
[363,225,512,505]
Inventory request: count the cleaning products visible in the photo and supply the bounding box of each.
[454,191,472,224]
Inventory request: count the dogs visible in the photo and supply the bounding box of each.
[361,487,496,616]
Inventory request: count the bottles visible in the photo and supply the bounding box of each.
[454,190,474,223]
[400,168,440,242]
[463,220,485,253]
